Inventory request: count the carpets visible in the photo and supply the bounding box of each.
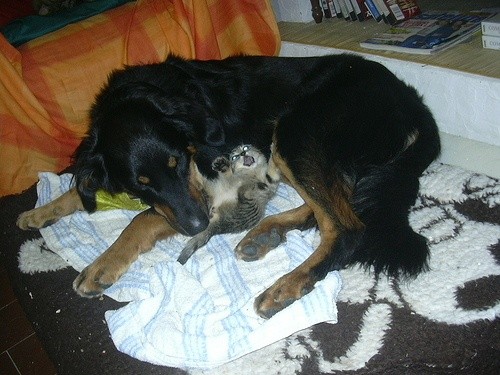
[0,158,500,375]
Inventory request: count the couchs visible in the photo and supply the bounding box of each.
[0,0,282,196]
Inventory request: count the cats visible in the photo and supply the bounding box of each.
[176,140,282,266]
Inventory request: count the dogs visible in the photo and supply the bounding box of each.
[15,51,442,319]
